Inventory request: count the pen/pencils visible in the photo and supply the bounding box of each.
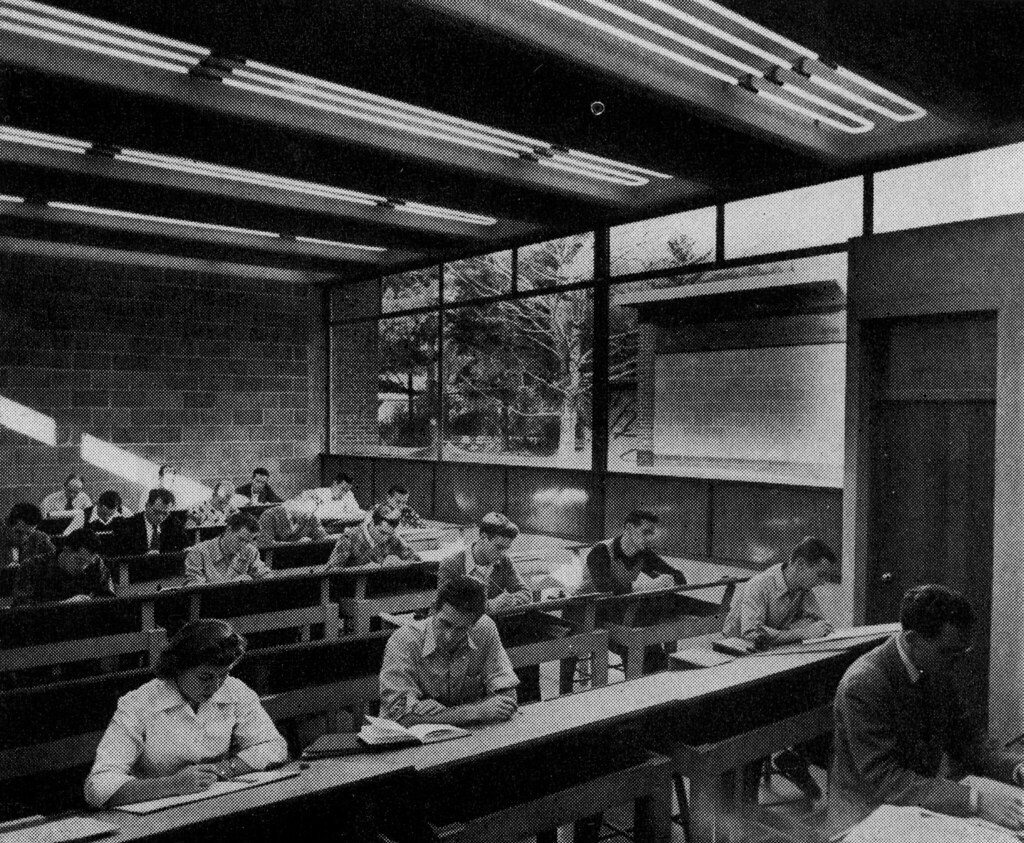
[810,612,838,635]
[652,570,662,577]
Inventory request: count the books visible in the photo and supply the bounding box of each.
[802,623,901,644]
[842,776,1024,843]
[0,716,470,843]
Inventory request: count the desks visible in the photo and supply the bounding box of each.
[0,500,903,843]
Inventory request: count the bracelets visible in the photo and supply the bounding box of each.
[229,759,236,776]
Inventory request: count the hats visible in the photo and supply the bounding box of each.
[480,513,519,537]
[377,502,401,520]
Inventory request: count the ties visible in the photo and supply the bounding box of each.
[150,525,161,551]
[65,498,74,510]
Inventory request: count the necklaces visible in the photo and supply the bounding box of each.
[313,475,358,516]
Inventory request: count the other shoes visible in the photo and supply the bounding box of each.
[773,750,824,799]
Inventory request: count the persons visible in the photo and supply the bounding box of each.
[13,529,115,603]
[380,576,519,726]
[237,467,282,502]
[438,512,530,606]
[82,618,289,805]
[368,487,426,528]
[565,511,687,673]
[42,465,190,555]
[828,584,1024,843]
[0,503,56,567]
[184,514,276,586]
[186,480,240,526]
[724,536,835,798]
[260,499,326,542]
[326,502,422,600]
